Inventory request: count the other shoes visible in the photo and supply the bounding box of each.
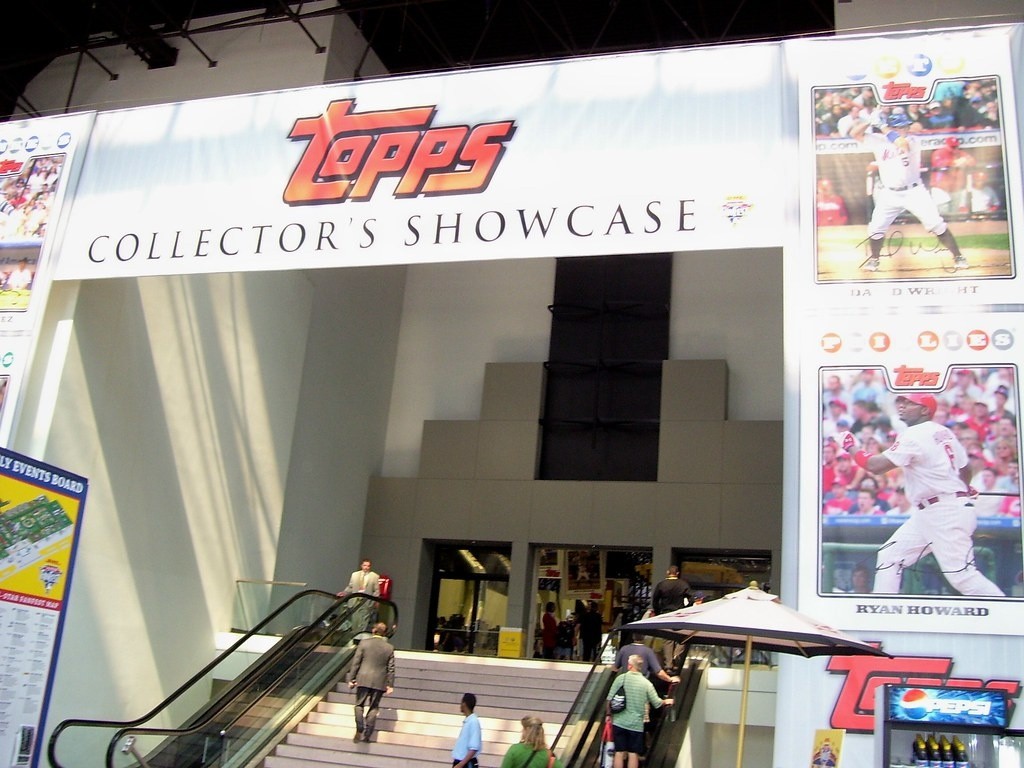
[364,737,369,742]
[354,731,361,743]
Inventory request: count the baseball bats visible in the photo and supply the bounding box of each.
[977,492,1019,496]
[825,91,863,110]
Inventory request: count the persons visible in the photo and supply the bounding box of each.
[542,602,560,658]
[612,634,680,760]
[653,565,696,674]
[438,616,455,651]
[348,623,395,744]
[501,715,563,768]
[605,655,674,768]
[815,80,1005,272]
[451,693,482,768]
[575,602,608,662]
[337,558,380,635]
[328,613,347,633]
[822,369,1021,596]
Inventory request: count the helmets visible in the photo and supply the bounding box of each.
[886,114,913,127]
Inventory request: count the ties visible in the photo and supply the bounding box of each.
[360,573,365,586]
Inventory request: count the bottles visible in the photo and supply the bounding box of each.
[912,733,970,768]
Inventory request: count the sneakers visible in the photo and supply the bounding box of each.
[863,258,879,272]
[954,255,969,269]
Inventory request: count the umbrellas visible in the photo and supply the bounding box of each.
[614,580,894,768]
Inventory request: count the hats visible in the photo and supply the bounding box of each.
[928,102,941,110]
[836,453,850,461]
[947,136,960,146]
[831,476,847,486]
[973,169,988,181]
[895,393,938,419]
[819,178,832,190]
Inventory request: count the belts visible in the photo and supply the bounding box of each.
[917,491,970,510]
[890,183,917,191]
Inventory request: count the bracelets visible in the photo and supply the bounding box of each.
[606,714,612,716]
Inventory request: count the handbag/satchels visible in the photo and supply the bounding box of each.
[610,675,626,713]
[599,721,615,768]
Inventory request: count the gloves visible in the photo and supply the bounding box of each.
[968,485,979,500]
[834,431,856,453]
[866,107,888,130]
